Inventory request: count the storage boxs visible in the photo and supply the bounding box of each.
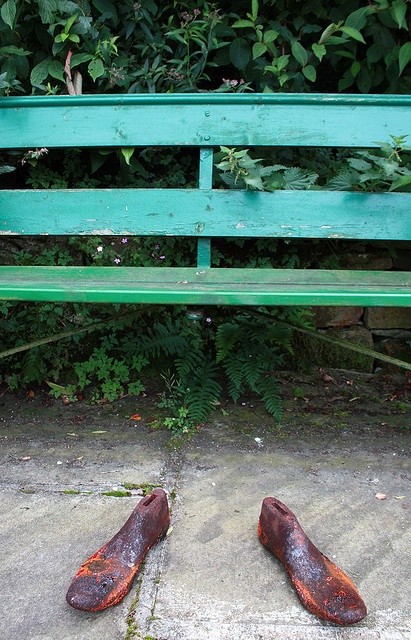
[0,94,410,308]
